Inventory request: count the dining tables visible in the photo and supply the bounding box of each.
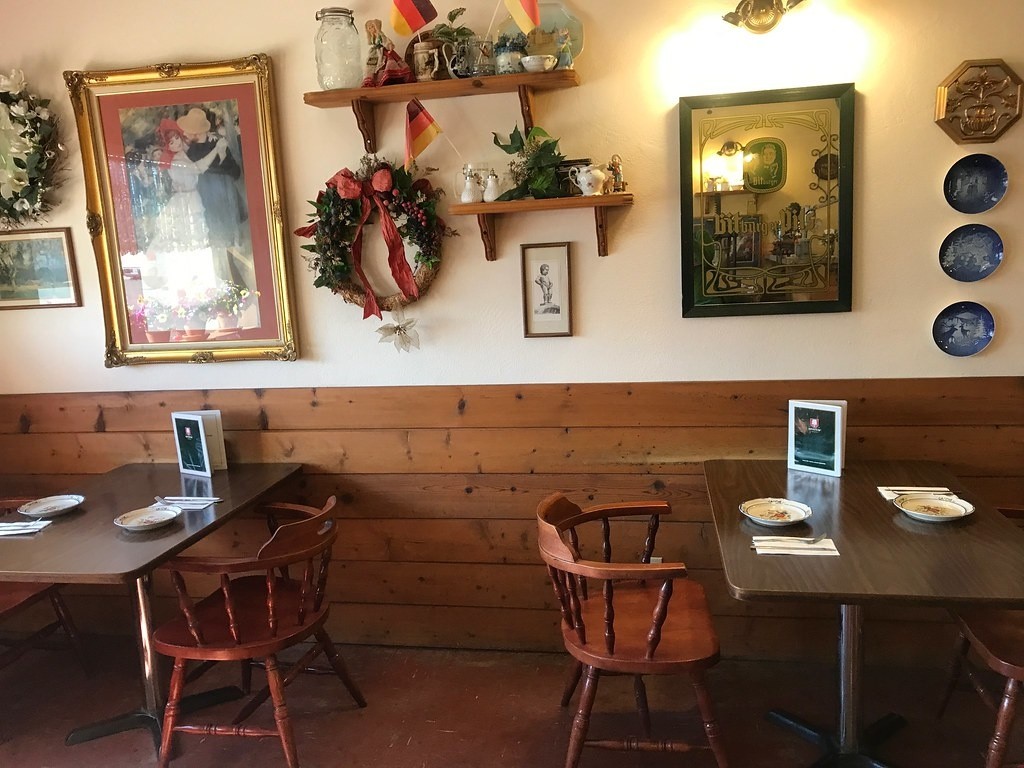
[0,459,304,761]
[705,456,1024,768]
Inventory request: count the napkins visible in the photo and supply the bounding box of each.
[0,521,53,535]
[753,536,840,556]
[877,486,953,501]
[150,497,220,510]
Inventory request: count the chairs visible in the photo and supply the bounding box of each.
[538,497,1024,768]
[0,488,368,768]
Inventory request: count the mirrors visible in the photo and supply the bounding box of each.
[675,84,854,317]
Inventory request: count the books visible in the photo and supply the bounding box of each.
[788,398,847,478]
[171,410,227,478]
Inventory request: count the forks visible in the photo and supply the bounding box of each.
[154,496,208,505]
[0,517,43,526]
[887,486,953,496]
[751,531,827,544]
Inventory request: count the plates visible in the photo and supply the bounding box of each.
[739,498,813,526]
[933,154,1009,359]
[17,494,86,517]
[403,30,453,81]
[893,494,975,521]
[113,506,183,530]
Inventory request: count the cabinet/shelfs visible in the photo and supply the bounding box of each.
[301,69,634,262]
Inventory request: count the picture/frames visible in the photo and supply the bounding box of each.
[519,242,573,339]
[0,228,81,310]
[65,53,297,366]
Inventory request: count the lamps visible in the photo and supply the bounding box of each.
[718,142,746,156]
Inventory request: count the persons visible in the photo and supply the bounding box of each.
[607,154,624,192]
[556,28,574,69]
[360,20,416,87]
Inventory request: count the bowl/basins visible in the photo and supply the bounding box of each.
[520,55,557,73]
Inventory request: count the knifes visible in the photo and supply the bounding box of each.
[0,528,40,531]
[886,490,951,492]
[165,499,224,501]
[750,546,836,551]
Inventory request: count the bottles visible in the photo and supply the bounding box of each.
[313,6,363,90]
[482,167,503,202]
[460,170,482,203]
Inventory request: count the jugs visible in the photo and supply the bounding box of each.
[568,163,607,196]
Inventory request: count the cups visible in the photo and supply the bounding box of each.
[413,37,495,82]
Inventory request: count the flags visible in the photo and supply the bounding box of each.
[504,0,541,35]
[404,96,442,174]
[390,0,438,35]
